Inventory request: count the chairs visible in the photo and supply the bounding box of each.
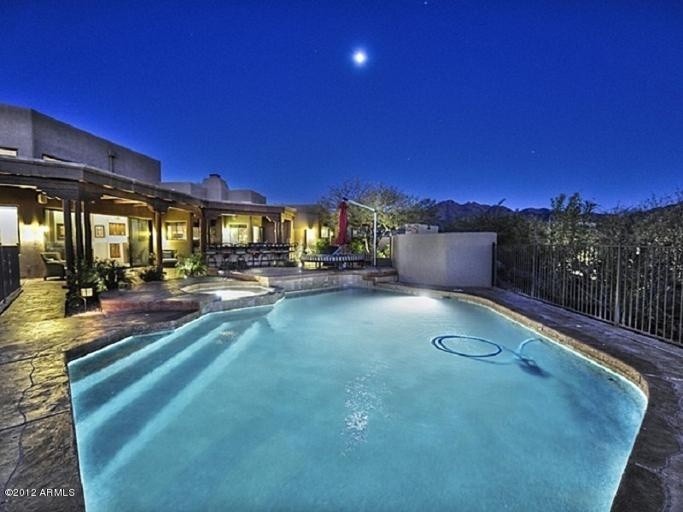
[207,241,298,269]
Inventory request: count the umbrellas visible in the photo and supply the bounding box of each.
[336,201,350,256]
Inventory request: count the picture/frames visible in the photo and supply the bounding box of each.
[56,223,64,240]
[94,225,105,238]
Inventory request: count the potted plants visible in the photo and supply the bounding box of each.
[173,252,208,279]
[97,257,128,290]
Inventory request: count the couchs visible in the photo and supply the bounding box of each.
[162,250,177,264]
[42,253,66,279]
